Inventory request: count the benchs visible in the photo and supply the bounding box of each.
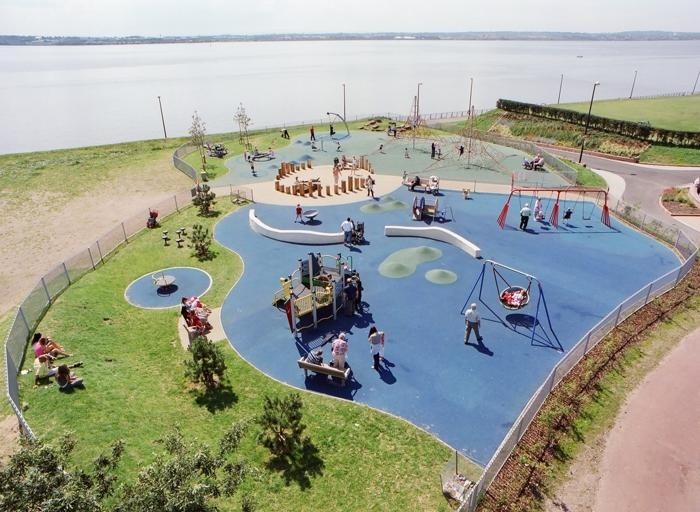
[297,357,351,387]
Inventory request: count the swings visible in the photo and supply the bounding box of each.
[534,190,554,221]
[563,192,582,222]
[583,192,601,221]
[488,265,533,311]
[519,190,533,215]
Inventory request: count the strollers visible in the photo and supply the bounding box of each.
[146,208,161,229]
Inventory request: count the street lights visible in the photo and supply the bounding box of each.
[577,81,601,166]
[557,72,564,104]
[416,81,423,128]
[629,69,639,100]
[467,78,474,120]
[157,95,168,146]
[341,82,346,125]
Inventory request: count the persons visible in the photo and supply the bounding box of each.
[306,350,323,366]
[289,154,379,200]
[249,125,353,153]
[331,332,349,371]
[502,285,532,306]
[402,169,444,193]
[293,205,309,218]
[339,217,368,245]
[279,246,367,310]
[520,189,573,230]
[180,296,212,336]
[464,303,483,344]
[520,152,555,172]
[369,326,380,370]
[374,140,471,163]
[31,332,83,390]
